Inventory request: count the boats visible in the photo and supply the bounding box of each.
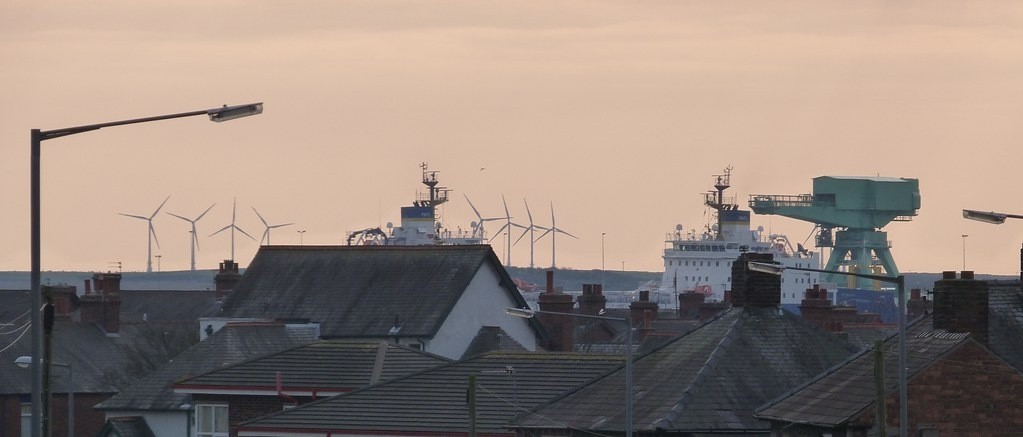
[660,163,908,325]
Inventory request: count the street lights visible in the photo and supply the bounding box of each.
[601,233,605,290]
[506,307,634,437]
[962,235,968,270]
[28,100,265,436]
